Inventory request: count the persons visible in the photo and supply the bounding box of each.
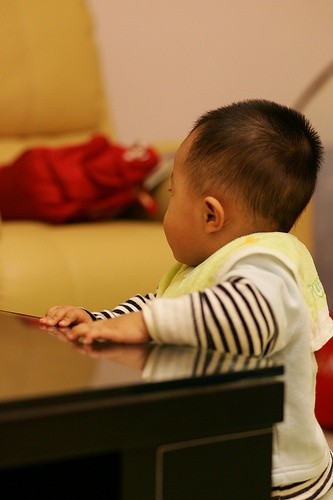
[37,97,333,500]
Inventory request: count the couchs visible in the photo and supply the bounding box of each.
[0,0,311,321]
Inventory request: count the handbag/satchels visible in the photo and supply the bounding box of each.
[0,134,160,225]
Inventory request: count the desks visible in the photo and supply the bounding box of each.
[0,309,285,500]
